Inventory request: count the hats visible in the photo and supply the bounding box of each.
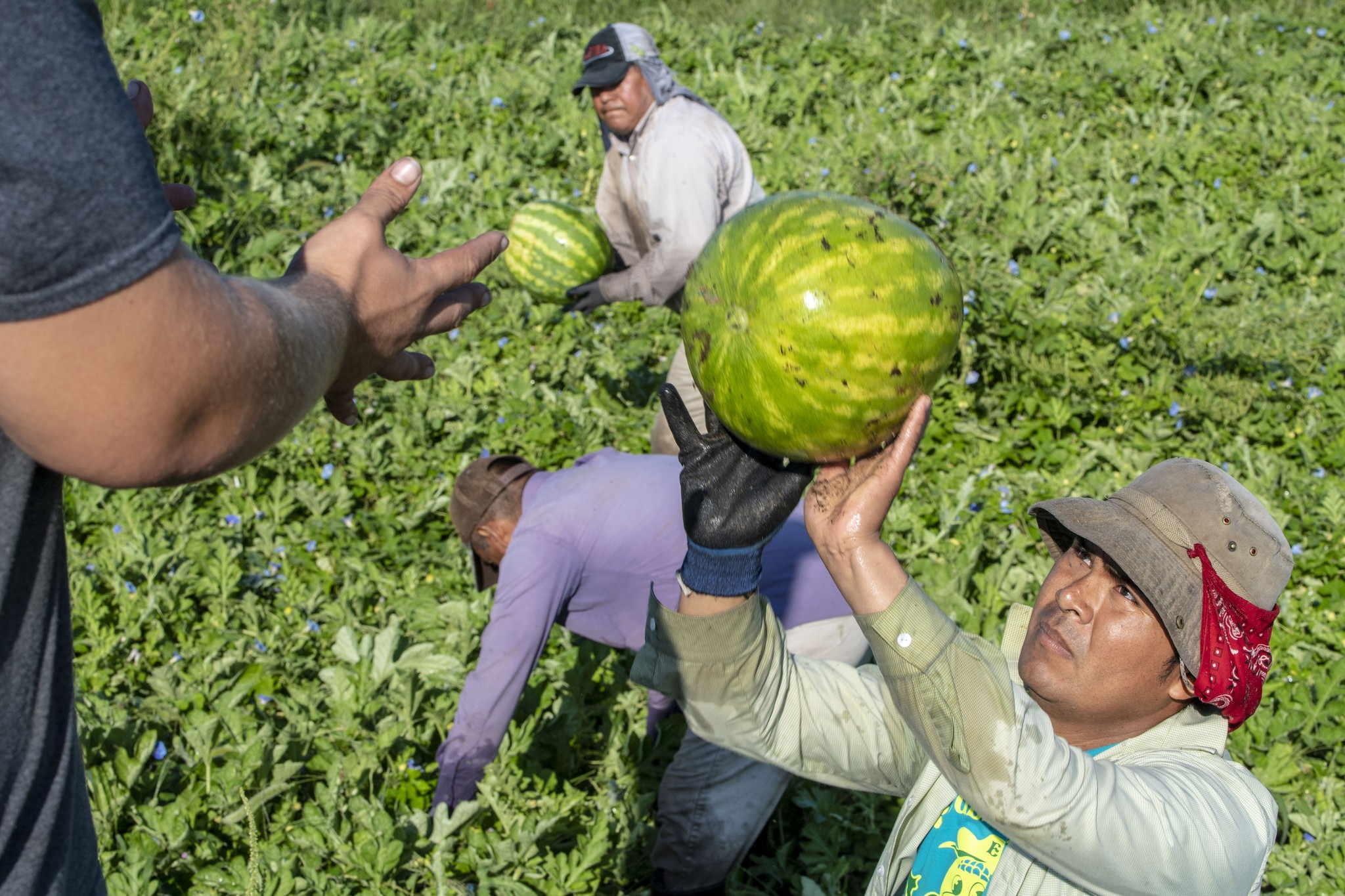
[450,455,547,593]
[572,22,660,95]
[1030,458,1294,686]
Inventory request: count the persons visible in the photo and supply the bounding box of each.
[429,447,892,896]
[0,1,510,896]
[560,22,771,456]
[659,383,1296,896]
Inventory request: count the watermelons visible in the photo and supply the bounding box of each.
[502,198,612,305]
[684,190,966,466]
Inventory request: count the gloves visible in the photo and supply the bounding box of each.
[562,279,608,319]
[658,383,819,596]
